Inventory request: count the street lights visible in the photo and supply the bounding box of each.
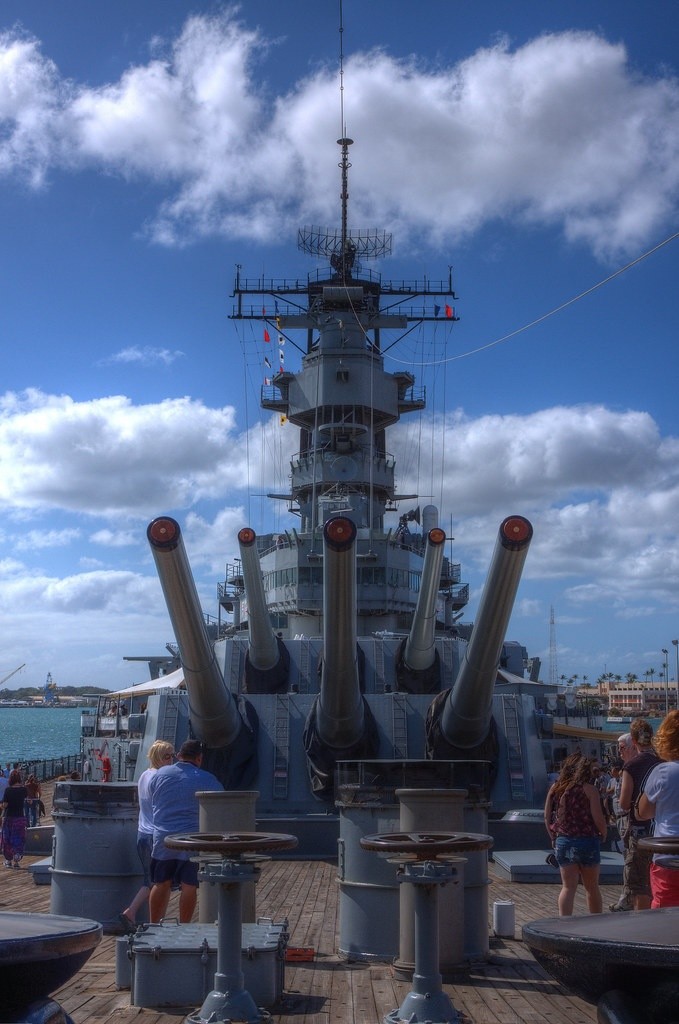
[662,649,668,715]
[672,640,679,710]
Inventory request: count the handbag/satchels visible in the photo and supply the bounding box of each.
[546,854,559,868]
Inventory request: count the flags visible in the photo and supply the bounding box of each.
[280,366,283,375]
[278,335,285,346]
[263,376,271,386]
[265,329,270,343]
[276,317,282,330]
[280,416,286,425]
[279,348,284,364]
[275,300,279,313]
[262,308,265,315]
[265,356,271,369]
[435,304,441,316]
[445,304,452,319]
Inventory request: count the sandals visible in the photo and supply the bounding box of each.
[609,904,626,912]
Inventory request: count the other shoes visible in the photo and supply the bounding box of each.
[4,862,12,867]
[13,863,19,868]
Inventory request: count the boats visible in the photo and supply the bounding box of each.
[71,2,611,859]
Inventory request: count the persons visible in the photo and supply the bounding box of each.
[609,717,665,912]
[105,704,122,716]
[0,762,46,869]
[544,752,608,916]
[148,738,225,923]
[118,740,175,932]
[592,762,630,837]
[634,710,679,909]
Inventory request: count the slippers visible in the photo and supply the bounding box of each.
[119,914,137,931]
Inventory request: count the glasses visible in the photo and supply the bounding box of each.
[619,745,636,751]
[164,753,174,760]
